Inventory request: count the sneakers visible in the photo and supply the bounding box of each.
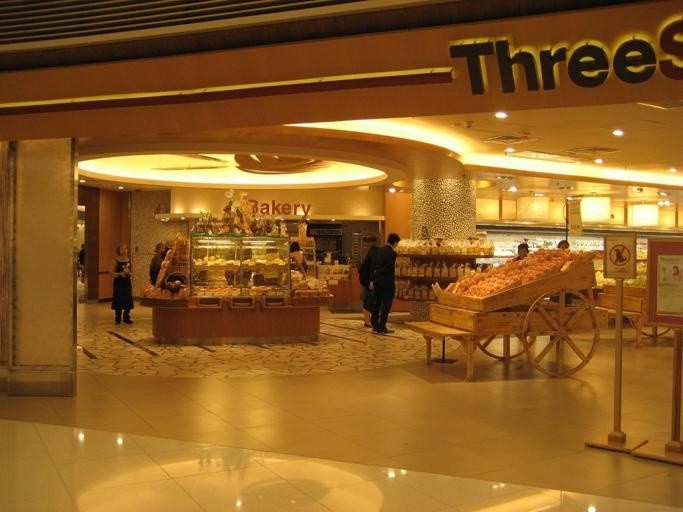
[364,322,395,334]
[123,318,133,324]
[115,316,122,322]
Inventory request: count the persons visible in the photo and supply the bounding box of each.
[359,246,381,327]
[107,242,136,326]
[368,234,401,333]
[160,239,171,260]
[512,241,529,260]
[289,241,306,272]
[148,242,164,286]
[78,243,86,283]
[550,239,574,342]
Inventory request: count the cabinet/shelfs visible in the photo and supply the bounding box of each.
[139,237,360,311]
[396,236,496,302]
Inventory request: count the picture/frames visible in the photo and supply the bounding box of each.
[646,236,683,331]
